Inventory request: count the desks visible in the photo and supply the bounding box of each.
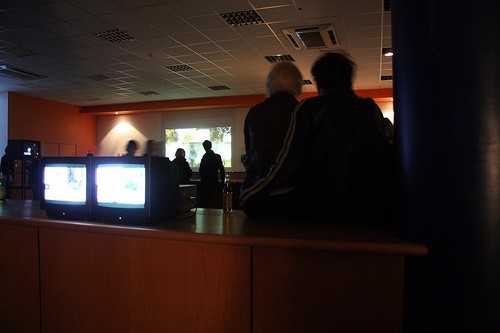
[189,178,245,184]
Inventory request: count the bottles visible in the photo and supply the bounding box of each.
[223,173,232,213]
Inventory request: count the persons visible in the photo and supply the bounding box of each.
[172,148,193,184]
[120,140,137,158]
[143,140,159,157]
[240,52,394,224]
[239,61,306,220]
[0,146,17,186]
[196,140,225,209]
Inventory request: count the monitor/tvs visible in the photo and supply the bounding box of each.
[8,139,40,159]
[39,156,91,221]
[92,155,180,225]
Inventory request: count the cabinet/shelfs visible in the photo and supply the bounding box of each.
[5,138,42,199]
[0,198,428,333]
